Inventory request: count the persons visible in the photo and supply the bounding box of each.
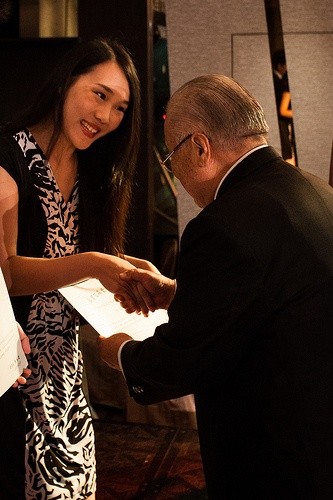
[97,68,333,500]
[10,317,32,388]
[0,39,156,500]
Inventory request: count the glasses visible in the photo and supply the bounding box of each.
[158,131,212,173]
